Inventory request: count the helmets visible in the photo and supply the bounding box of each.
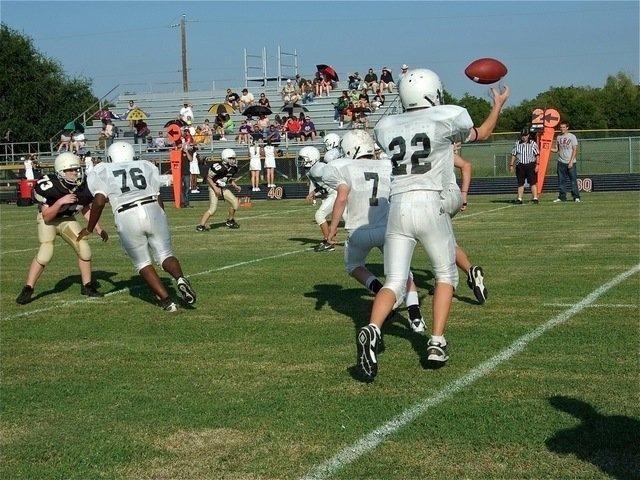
[298,146,320,168]
[337,129,376,159]
[222,148,238,168]
[54,152,84,193]
[106,141,138,163]
[398,69,444,112]
[323,133,339,150]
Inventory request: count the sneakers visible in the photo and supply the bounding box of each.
[81,282,105,298]
[356,327,379,379]
[268,185,270,187]
[467,266,487,304]
[574,198,580,203]
[513,200,523,204]
[425,339,449,362]
[532,200,538,204]
[252,188,256,191]
[176,277,195,305]
[271,184,276,187]
[196,224,209,233]
[314,242,335,252]
[191,190,200,193]
[16,285,33,304]
[553,199,568,203]
[408,315,427,333]
[256,188,261,191]
[225,219,239,228]
[159,302,178,313]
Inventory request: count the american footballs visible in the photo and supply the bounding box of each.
[465,59,507,84]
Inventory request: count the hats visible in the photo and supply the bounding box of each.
[401,64,408,69]
[304,116,311,120]
[241,89,248,92]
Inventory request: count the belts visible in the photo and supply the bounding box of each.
[118,200,157,213]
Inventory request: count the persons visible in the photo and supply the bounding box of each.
[15,139,201,311]
[194,141,279,232]
[550,121,582,202]
[510,130,540,204]
[57,100,182,153]
[179,66,411,140]
[298,129,488,331]
[361,69,510,380]
